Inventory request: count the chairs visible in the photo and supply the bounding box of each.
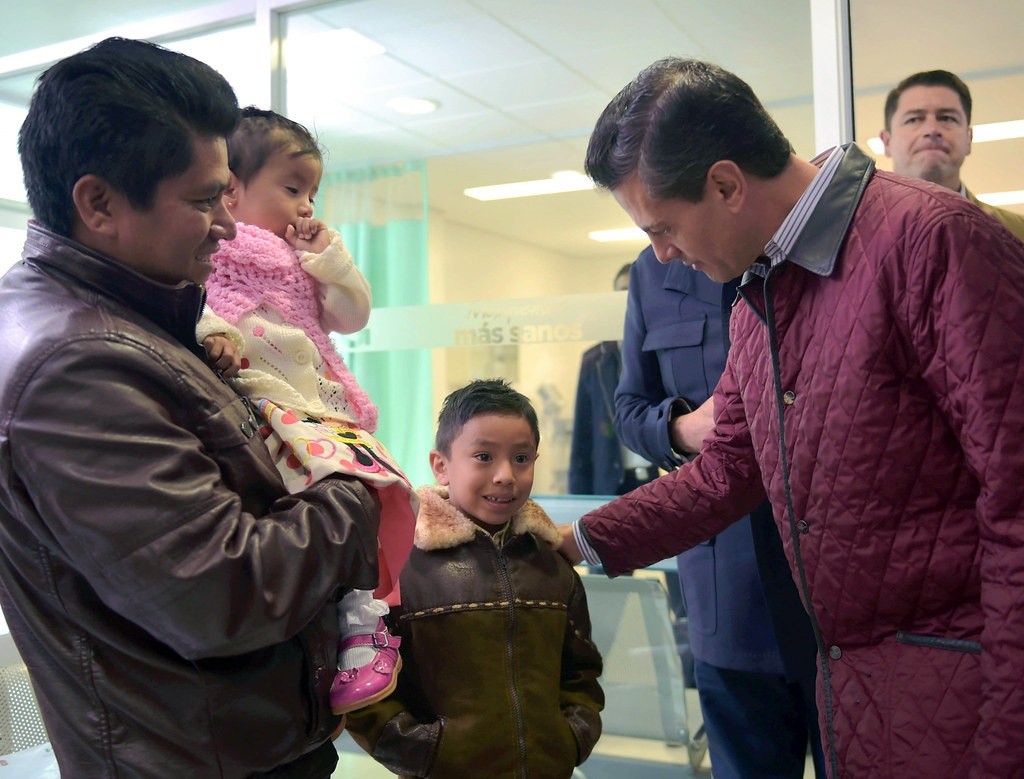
[573,565,709,779]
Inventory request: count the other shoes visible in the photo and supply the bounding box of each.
[328,616,402,715]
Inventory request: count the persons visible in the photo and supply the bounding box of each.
[0,35,383,779]
[197,67,1024,779]
[554,56,1023,779]
